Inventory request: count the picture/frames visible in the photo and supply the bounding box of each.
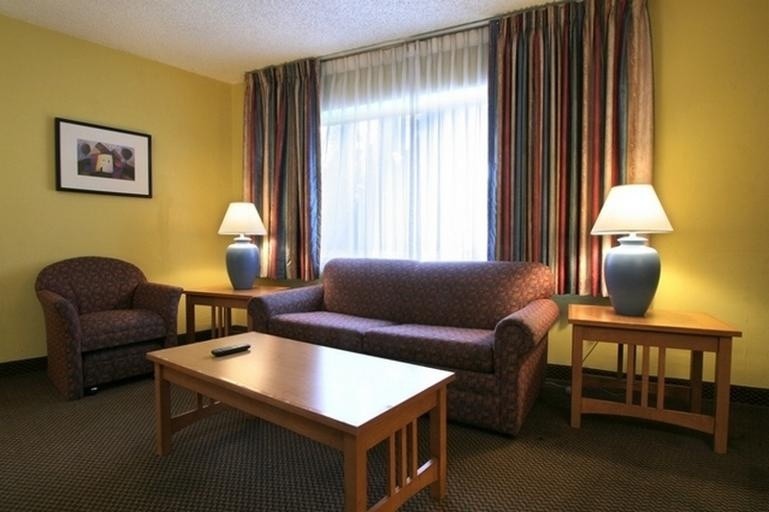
[55,117,151,198]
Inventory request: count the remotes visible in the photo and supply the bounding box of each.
[212,343,250,356]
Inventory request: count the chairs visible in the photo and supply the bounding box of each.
[34,256,183,401]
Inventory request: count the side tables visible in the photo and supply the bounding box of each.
[567,305,743,455]
[183,285,292,343]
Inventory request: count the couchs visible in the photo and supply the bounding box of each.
[247,258,559,436]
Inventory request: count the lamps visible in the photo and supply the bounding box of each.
[590,183,674,316]
[216,202,267,290]
